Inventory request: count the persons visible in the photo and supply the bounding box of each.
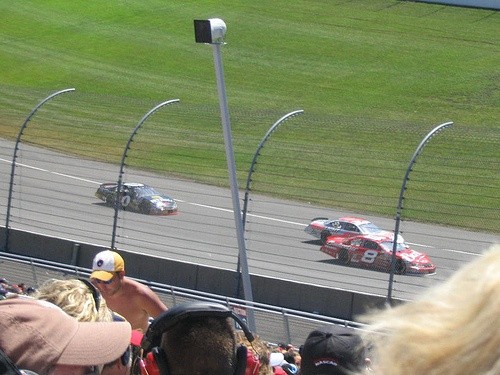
[357,244,500,375]
[0,277,41,303]
[35,277,114,375]
[90,250,168,335]
[300,327,373,375]
[157,302,239,375]
[100,310,133,375]
[231,329,302,375]
[0,295,132,375]
[0,348,40,375]
[129,313,158,375]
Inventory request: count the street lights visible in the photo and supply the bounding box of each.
[194,18,256,333]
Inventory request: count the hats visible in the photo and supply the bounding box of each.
[271,352,284,367]
[0,292,131,375]
[90,250,124,282]
[299,327,365,375]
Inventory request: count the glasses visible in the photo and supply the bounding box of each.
[78,277,100,311]
[94,274,119,285]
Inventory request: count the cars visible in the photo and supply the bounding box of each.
[320,233,437,277]
[95,182,178,215]
[304,215,407,243]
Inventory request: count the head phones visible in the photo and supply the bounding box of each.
[133,302,260,375]
